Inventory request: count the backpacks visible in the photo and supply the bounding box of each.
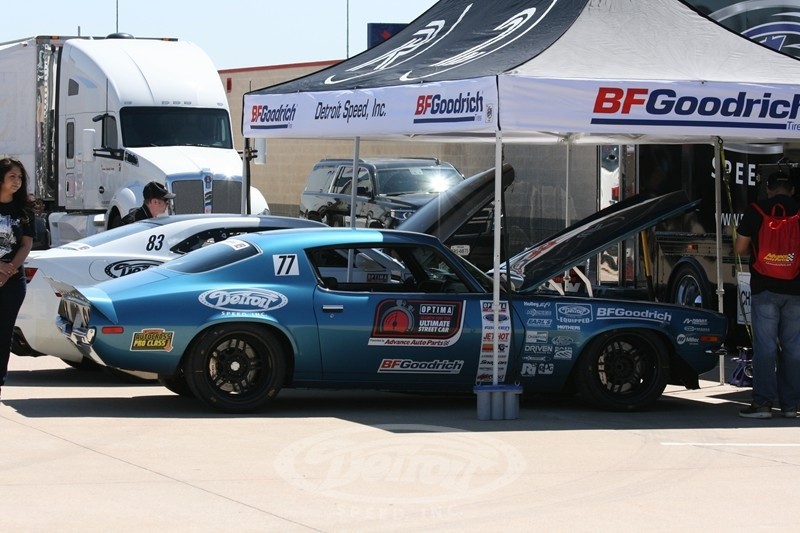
[748,203,800,279]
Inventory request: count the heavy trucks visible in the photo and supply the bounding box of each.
[1,33,273,249]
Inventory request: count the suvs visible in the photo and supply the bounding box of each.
[296,158,510,271]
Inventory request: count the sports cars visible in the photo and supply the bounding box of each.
[20,162,736,413]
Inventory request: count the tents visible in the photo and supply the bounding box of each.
[241,0,800,390]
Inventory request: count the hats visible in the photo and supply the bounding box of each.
[143,182,176,200]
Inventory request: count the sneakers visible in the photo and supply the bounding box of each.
[779,409,796,417]
[740,400,771,418]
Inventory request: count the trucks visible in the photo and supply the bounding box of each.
[602,141,751,312]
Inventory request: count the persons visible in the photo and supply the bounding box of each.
[735,170,800,419]
[117,181,176,226]
[0,157,36,399]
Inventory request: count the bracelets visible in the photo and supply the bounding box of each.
[9,262,19,274]
